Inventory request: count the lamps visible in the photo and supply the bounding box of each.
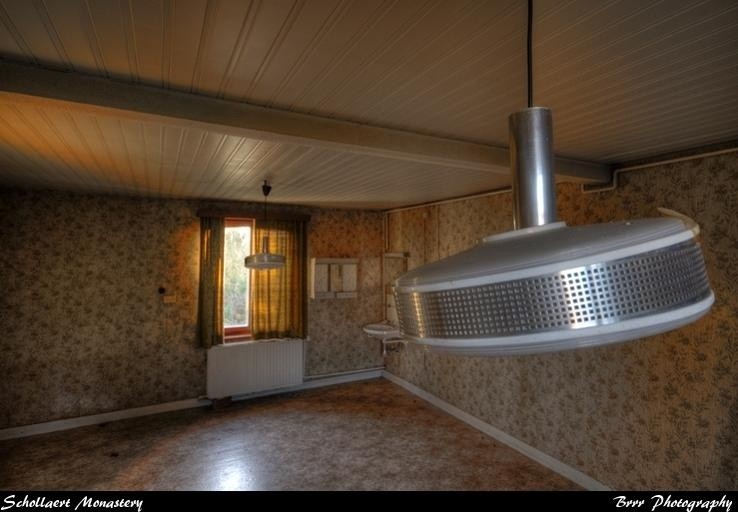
[244,179,285,269]
[391,0,717,357]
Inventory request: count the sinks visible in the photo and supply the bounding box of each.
[363,324,397,339]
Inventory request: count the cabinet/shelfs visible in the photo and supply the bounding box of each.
[310,257,360,300]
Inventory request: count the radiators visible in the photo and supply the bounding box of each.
[205,337,303,400]
